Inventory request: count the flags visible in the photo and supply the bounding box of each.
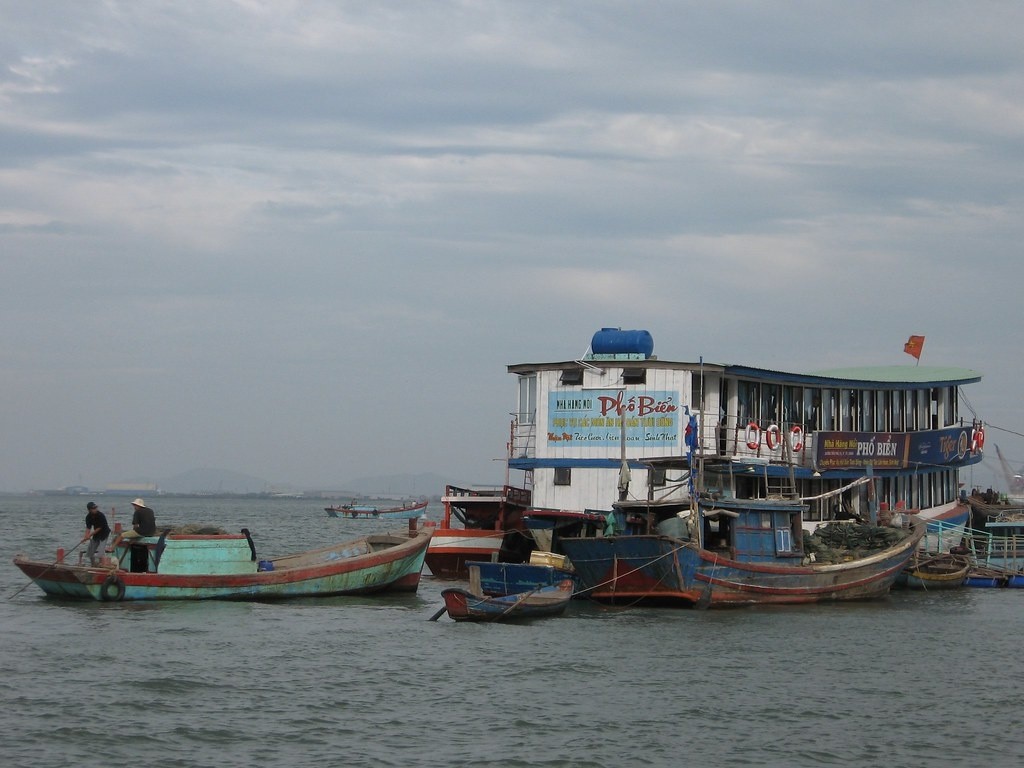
[904,334,925,359]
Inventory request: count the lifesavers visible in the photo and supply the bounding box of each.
[101,576,126,603]
[766,425,781,450]
[745,423,760,449]
[971,428,986,455]
[789,426,803,453]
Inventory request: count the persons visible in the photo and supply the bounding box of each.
[80,502,111,567]
[411,500,417,508]
[104,498,156,552]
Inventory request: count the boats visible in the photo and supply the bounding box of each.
[12,518,437,603]
[555,460,925,609]
[324,500,429,519]
[425,352,986,583]
[464,560,582,601]
[967,495,1024,534]
[441,577,576,623]
[894,547,972,590]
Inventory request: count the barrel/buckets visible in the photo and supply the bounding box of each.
[1005,575,1024,588]
[962,578,1001,588]
[259,559,273,572]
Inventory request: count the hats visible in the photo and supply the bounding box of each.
[87,502,98,509]
[131,498,146,508]
